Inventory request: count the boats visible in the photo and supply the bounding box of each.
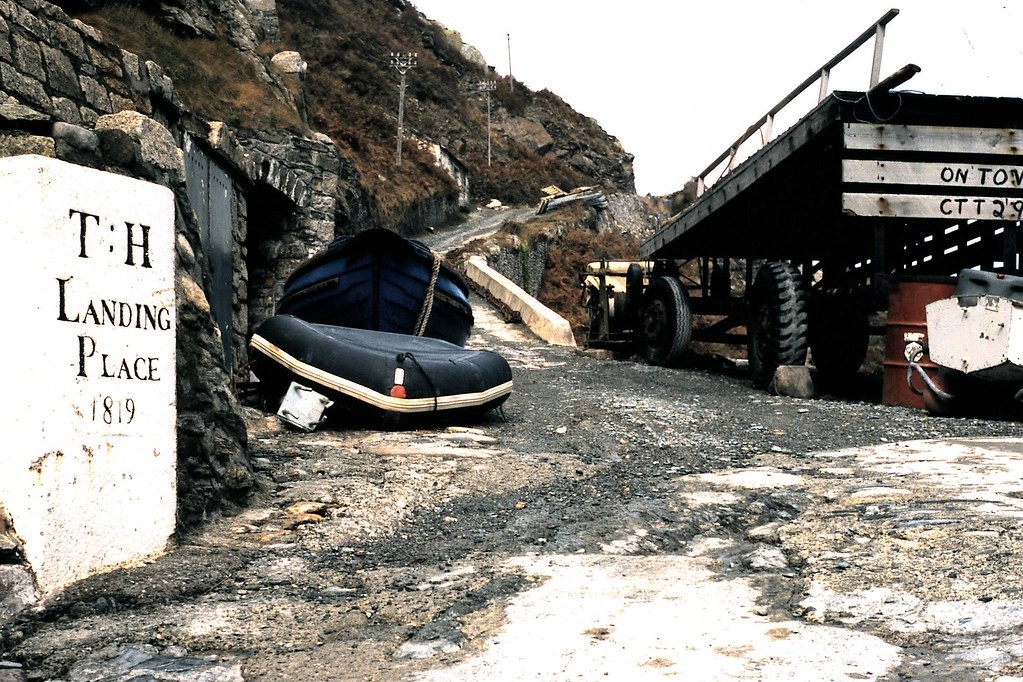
[279,222,473,349]
[245,314,516,432]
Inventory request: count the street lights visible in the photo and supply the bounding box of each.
[389,52,418,166]
[478,81,497,164]
